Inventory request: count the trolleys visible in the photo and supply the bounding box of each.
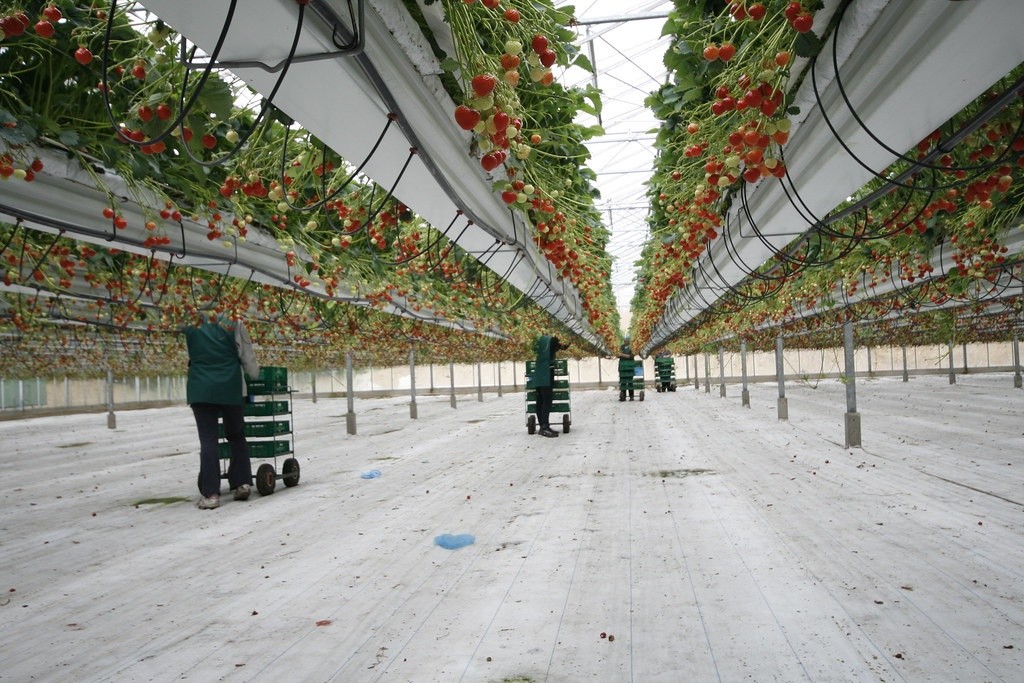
[619,360,645,401]
[217,366,300,497]
[525,359,572,435]
[655,358,677,392]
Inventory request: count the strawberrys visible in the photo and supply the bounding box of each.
[0,0,1024,360]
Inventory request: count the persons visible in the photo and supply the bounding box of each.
[183,312,260,509]
[533,334,571,437]
[616,335,634,401]
[656,347,673,392]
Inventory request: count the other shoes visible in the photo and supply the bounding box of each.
[539,427,559,437]
[630,396,634,401]
[620,397,625,400]
[668,389,673,391]
[662,388,665,392]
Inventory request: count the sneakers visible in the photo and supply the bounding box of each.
[233,483,251,500]
[198,492,219,509]
